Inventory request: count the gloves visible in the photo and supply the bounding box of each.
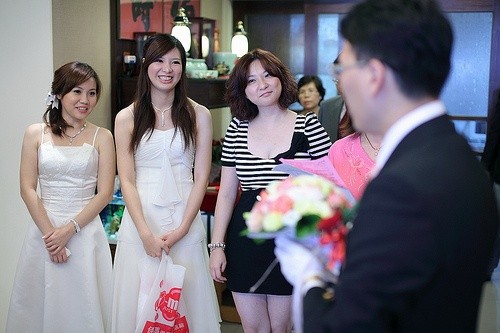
[275,237,326,294]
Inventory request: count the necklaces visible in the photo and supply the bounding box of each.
[152,102,173,127]
[364,133,382,156]
[63,121,87,143]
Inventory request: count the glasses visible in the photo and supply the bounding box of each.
[331,49,373,80]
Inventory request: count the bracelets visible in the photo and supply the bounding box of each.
[207,241,226,252]
[71,219,81,233]
[302,275,323,286]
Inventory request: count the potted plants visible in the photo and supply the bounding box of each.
[131,0,158,74]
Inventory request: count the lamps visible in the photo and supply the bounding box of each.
[231,20,249,58]
[201,32,210,57]
[169,5,191,55]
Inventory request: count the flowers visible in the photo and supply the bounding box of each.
[246,180,354,272]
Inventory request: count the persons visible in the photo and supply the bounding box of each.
[295,75,327,118]
[329,129,383,204]
[6,61,117,333]
[275,1,500,333]
[209,50,333,333]
[106,32,224,333]
[318,55,356,144]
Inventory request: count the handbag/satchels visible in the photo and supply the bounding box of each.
[136,252,194,333]
